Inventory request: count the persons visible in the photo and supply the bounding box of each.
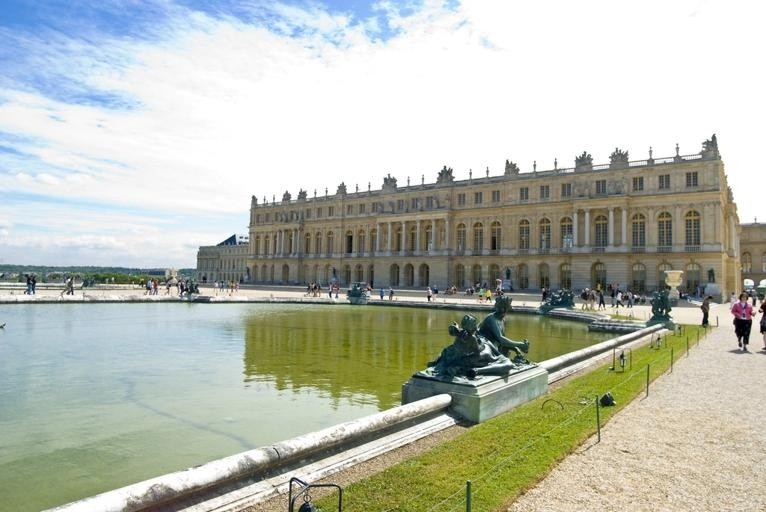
[478,291,531,361]
[59,276,70,295]
[699,294,712,325]
[758,298,766,350]
[730,292,755,352]
[380,287,384,299]
[426,276,504,304]
[143,274,239,295]
[650,289,673,319]
[302,279,341,299]
[366,283,371,296]
[66,277,75,295]
[538,278,653,311]
[388,286,394,299]
[730,293,737,310]
[442,311,512,377]
[24,274,32,295]
[31,277,36,294]
